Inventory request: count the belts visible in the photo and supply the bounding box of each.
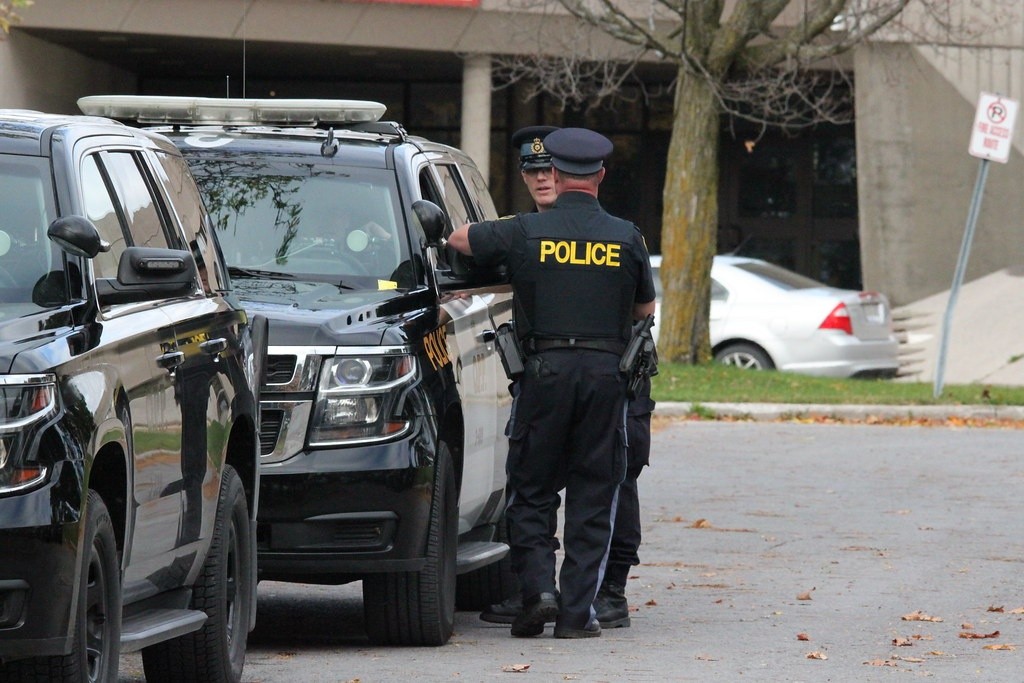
[534,337,627,356]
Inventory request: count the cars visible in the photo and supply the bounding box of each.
[649,255,899,381]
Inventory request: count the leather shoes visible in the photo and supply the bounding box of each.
[511,590,558,637]
[591,579,629,628]
[479,588,560,624]
[555,619,601,637]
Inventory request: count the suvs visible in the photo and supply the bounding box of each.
[77,94,513,646]
[0,108,270,683]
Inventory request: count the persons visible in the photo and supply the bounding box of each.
[448,126,659,638]
[328,212,394,254]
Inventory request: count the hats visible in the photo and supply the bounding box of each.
[510,125,614,175]
